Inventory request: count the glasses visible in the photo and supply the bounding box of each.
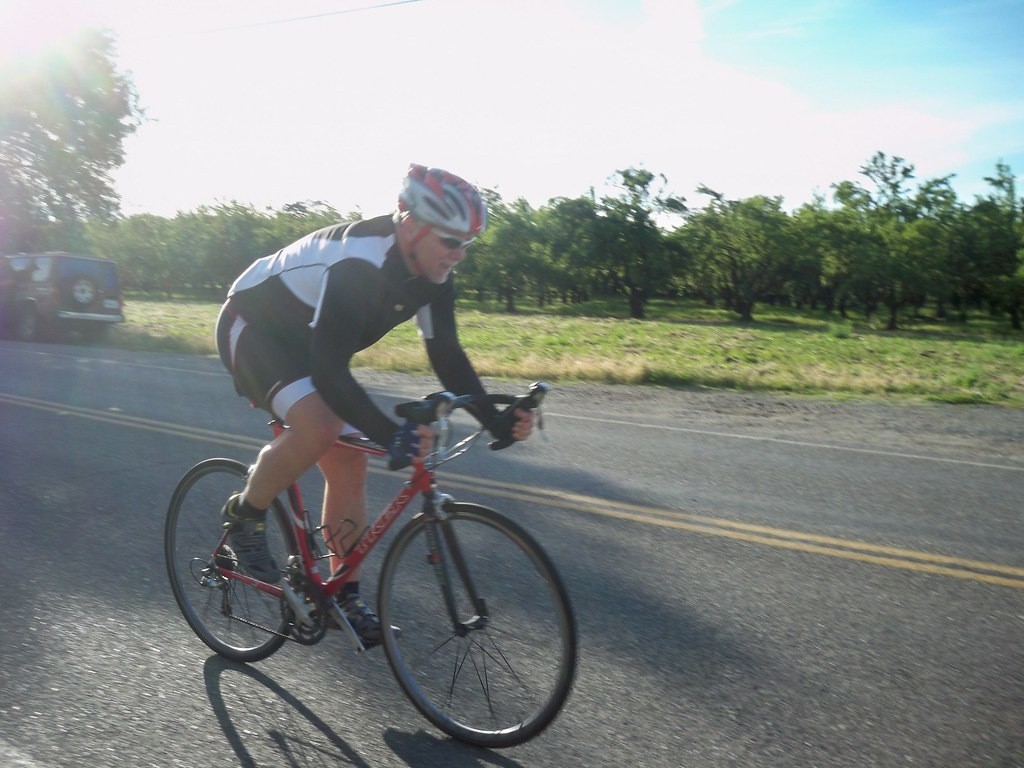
[438,235,472,250]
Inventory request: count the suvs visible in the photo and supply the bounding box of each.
[0,251,127,344]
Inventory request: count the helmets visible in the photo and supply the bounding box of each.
[398,162,487,234]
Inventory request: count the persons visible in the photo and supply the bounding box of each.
[216,165,535,638]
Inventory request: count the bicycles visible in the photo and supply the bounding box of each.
[160,379,579,752]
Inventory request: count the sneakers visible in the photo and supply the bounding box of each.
[220,491,282,584]
[321,592,402,643]
